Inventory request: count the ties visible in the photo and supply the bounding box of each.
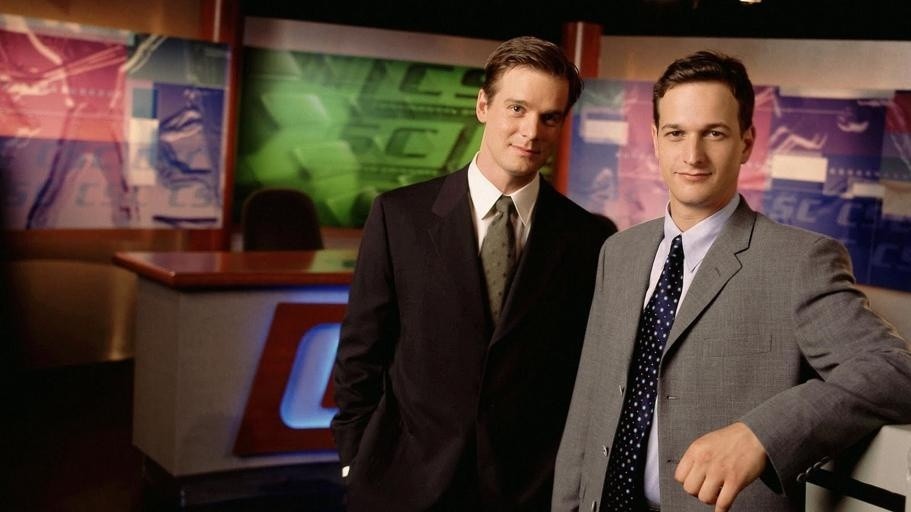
[600,236,683,512]
[481,196,517,333]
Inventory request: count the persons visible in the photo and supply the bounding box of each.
[326,32,618,511]
[546,46,910,510]
